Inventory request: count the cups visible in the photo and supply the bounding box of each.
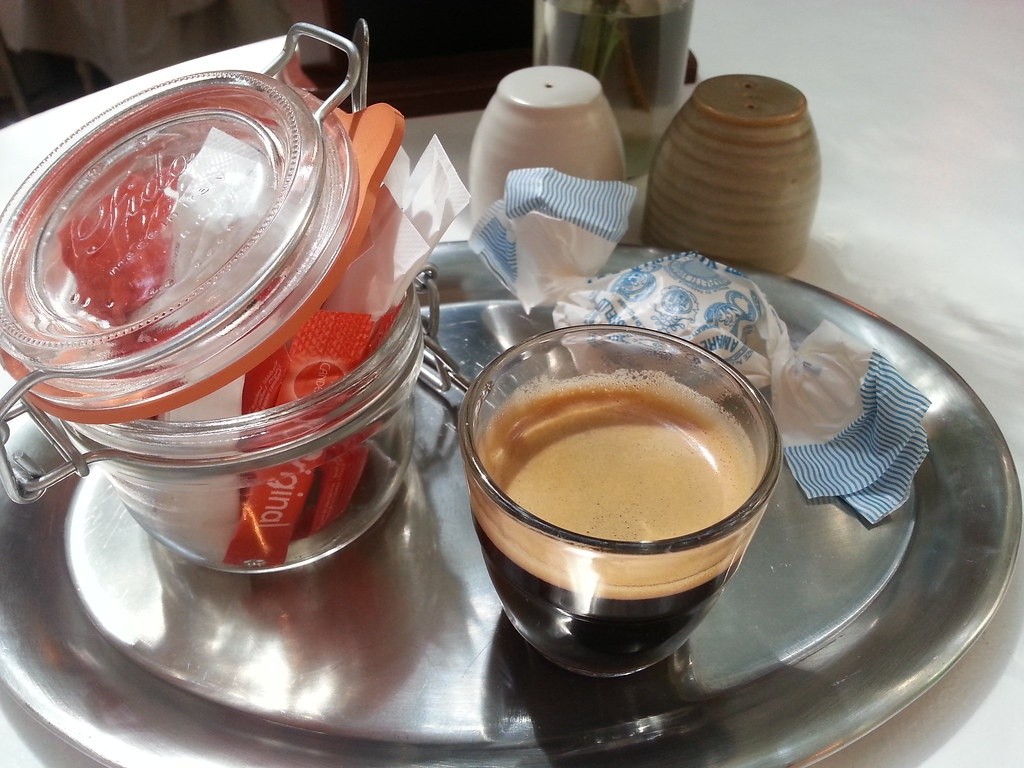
[458,323,783,678]
[533,0,695,181]
[58,283,425,575]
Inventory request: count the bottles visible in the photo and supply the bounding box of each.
[467,61,826,275]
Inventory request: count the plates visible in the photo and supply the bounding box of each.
[0,240,1023,768]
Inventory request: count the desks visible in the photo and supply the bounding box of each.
[0,111,1024,768]
